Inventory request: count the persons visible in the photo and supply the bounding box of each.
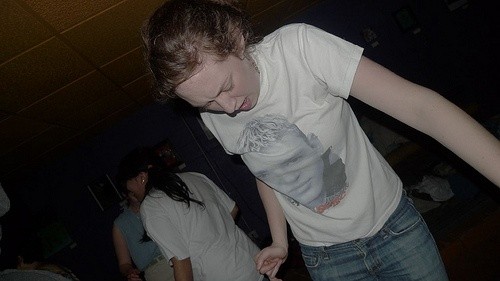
[138,1,499,281]
[119,154,271,281]
[111,195,175,280]
[16,243,79,281]
